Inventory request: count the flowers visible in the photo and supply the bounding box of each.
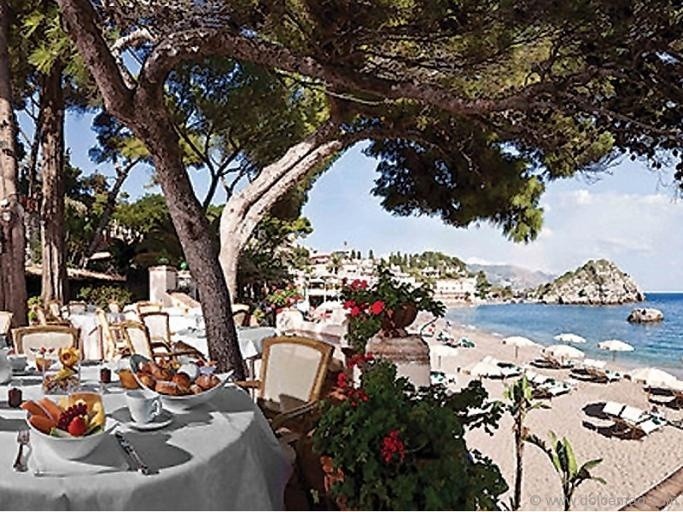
[307,354,509,509]
[343,260,445,320]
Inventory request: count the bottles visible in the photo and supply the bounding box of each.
[0,348,14,384]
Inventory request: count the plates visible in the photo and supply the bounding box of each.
[111,406,175,428]
[10,368,30,376]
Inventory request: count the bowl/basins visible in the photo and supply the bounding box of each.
[22,409,119,461]
[129,353,235,413]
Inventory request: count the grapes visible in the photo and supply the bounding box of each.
[55,402,88,431]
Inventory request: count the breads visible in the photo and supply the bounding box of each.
[119,358,222,396]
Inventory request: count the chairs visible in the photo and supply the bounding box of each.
[499,351,621,397]
[602,395,664,442]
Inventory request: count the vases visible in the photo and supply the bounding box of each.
[373,301,417,337]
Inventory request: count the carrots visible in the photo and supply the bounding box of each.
[18,397,64,432]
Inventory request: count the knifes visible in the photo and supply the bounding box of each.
[115,431,150,475]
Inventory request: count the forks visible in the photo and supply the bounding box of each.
[11,423,30,471]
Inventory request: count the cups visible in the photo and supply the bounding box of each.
[7,355,28,371]
[123,389,162,423]
[35,346,79,374]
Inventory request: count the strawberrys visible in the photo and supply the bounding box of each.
[67,414,88,436]
